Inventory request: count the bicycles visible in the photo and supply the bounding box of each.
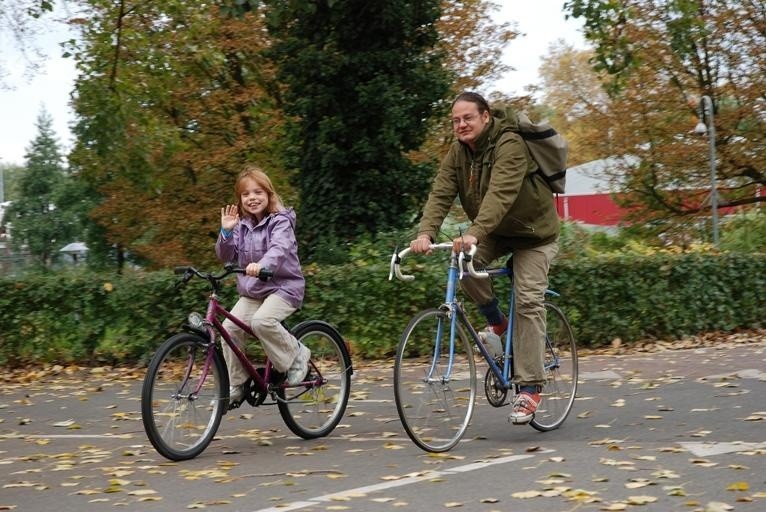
[140,263,354,462]
[389,228,579,454]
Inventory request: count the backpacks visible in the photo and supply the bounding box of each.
[490,112,567,192]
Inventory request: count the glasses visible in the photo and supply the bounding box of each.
[453,114,479,125]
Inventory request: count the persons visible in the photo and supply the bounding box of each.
[210,166,312,409]
[410,92,560,424]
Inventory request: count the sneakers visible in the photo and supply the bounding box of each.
[210,385,243,406]
[509,391,542,423]
[481,313,508,338]
[287,341,311,387]
